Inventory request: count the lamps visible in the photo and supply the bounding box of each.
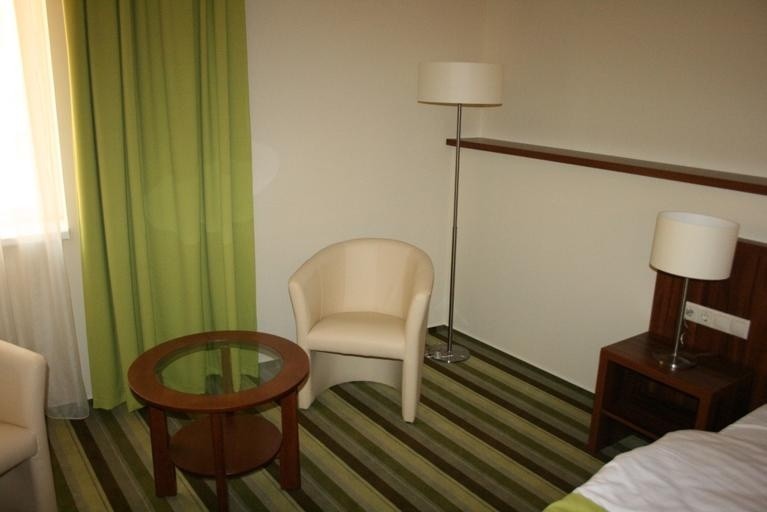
[416,56,502,367]
[647,201,745,376]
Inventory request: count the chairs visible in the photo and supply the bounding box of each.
[282,233,436,426]
[0,339,65,510]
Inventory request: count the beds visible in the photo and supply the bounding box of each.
[529,235,767,512]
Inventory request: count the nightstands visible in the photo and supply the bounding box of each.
[592,335,753,451]
